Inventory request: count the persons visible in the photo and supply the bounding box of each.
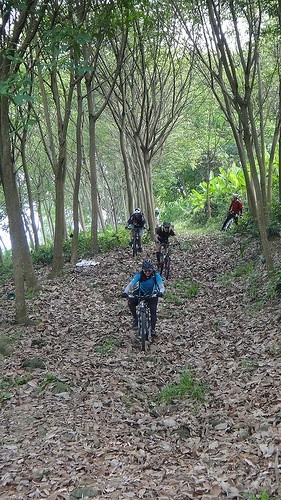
[125,208,147,252]
[122,258,164,336]
[221,194,242,231]
[154,222,180,263]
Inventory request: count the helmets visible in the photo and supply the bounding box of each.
[134,209,141,214]
[163,221,171,227]
[142,260,154,269]
[233,194,238,198]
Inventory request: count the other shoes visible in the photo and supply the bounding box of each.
[157,256,160,262]
[131,318,138,327]
[151,330,157,337]
[139,247,142,252]
[129,241,133,246]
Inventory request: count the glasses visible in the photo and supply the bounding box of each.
[145,270,152,272]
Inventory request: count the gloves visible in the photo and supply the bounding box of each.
[122,293,128,298]
[158,292,162,297]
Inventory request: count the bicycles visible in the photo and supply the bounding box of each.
[154,242,179,280]
[127,293,158,352]
[126,226,146,258]
